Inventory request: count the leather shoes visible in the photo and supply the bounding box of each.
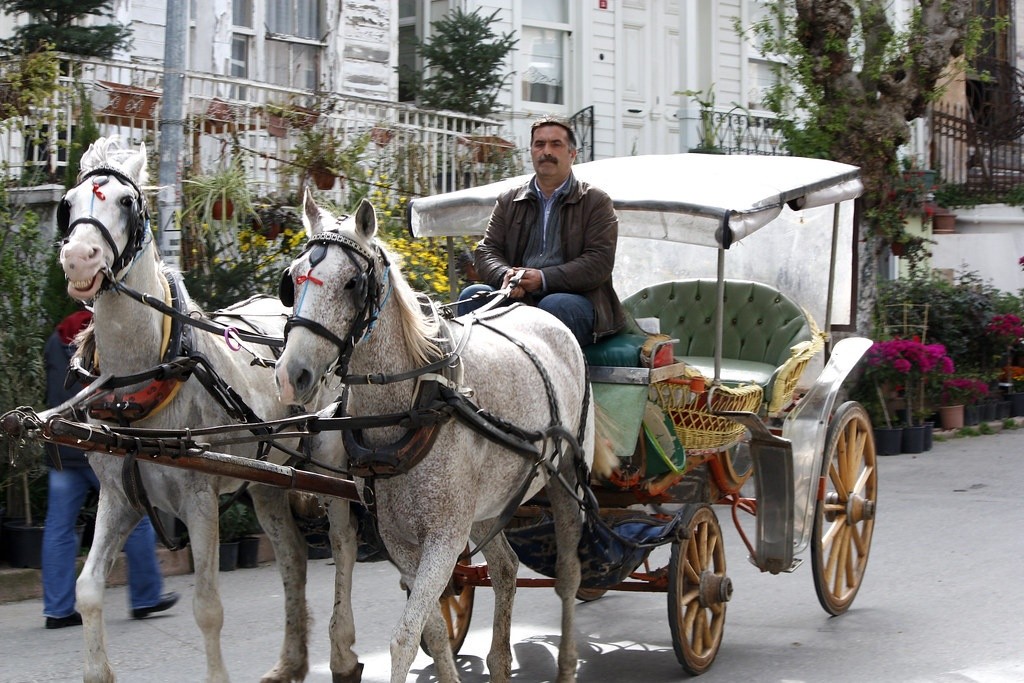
[134,592,179,618]
[46,613,83,629]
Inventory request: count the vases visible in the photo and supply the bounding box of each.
[874,421,933,456]
[889,241,906,256]
[903,169,937,191]
[92,80,162,130]
[205,97,236,122]
[939,392,1024,430]
[933,212,957,230]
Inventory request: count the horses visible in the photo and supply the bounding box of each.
[60,138,364,683]
[273,186,594,682]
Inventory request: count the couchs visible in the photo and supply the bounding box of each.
[618,278,827,418]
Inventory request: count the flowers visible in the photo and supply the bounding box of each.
[862,339,955,428]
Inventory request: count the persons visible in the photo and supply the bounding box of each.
[456,119,631,347]
[41,301,179,633]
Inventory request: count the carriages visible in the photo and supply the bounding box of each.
[1,130,878,683]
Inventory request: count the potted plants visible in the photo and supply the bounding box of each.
[0,36,80,121]
[263,79,343,139]
[177,147,301,240]
[369,116,401,148]
[459,130,525,182]
[272,132,366,191]
